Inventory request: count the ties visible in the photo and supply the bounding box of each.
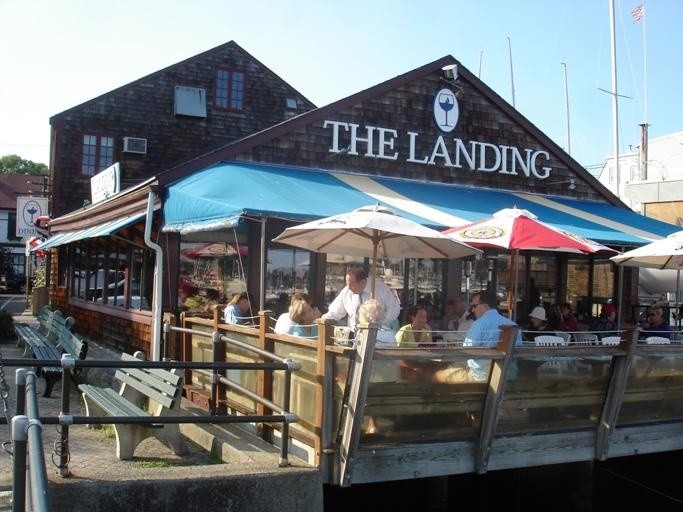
[353,295,361,336]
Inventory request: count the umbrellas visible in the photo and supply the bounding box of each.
[608,228,683,276]
[440,205,625,323]
[269,201,486,300]
[176,242,249,289]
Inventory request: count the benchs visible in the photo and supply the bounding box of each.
[15,305,96,395]
[78,351,191,459]
[351,372,680,428]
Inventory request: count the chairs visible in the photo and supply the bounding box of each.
[412,322,682,373]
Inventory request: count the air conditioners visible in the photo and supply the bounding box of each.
[120,135,148,156]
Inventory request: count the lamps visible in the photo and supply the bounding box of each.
[544,175,578,190]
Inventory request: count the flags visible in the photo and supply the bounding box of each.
[630,3,647,24]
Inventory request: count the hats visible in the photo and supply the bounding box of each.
[528,306,547,321]
[600,304,616,312]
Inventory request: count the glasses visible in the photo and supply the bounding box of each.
[415,304,426,309]
[473,304,479,308]
[646,312,655,316]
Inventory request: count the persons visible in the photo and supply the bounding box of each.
[221,256,683,389]
